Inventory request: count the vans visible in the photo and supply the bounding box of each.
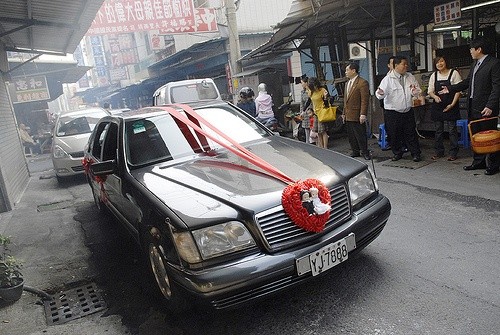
[152,77,223,106]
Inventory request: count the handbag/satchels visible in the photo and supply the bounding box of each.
[315,99,337,122]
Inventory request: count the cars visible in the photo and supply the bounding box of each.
[109,108,146,133]
[84,99,392,317]
[51,106,113,184]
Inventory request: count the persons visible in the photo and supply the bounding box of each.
[438,38,500,175]
[299,77,331,149]
[18,124,41,157]
[375,55,422,162]
[427,54,462,161]
[301,186,332,215]
[342,63,371,160]
[237,83,274,123]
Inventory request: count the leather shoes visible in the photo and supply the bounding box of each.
[463,162,486,170]
[484,167,500,175]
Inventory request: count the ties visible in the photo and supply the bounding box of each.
[347,81,351,96]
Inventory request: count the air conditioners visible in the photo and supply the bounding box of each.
[348,42,366,60]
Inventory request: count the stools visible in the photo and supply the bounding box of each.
[456,119,472,150]
[377,123,391,150]
[24,145,32,159]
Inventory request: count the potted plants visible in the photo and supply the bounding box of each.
[0,236,25,305]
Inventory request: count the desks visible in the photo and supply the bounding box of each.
[413,97,469,137]
[35,134,54,154]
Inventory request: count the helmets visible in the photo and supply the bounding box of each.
[239,87,254,98]
[257,83,268,92]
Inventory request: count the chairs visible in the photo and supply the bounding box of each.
[125,135,158,164]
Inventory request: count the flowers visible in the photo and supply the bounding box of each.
[282,179,331,234]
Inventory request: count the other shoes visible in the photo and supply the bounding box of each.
[431,154,444,160]
[413,153,421,162]
[362,152,371,160]
[392,154,402,161]
[447,154,457,161]
